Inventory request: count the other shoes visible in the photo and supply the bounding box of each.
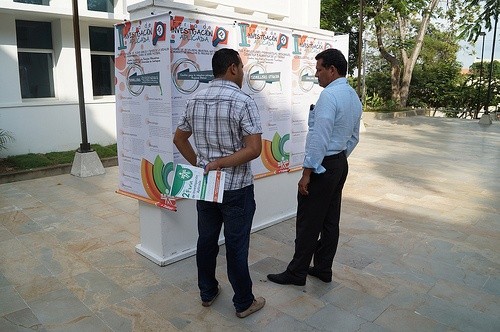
[236,296,266,318]
[202,283,223,307]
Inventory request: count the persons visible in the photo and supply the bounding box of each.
[267,49,362,286]
[174,48,265,317]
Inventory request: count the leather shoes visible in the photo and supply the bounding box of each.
[267,270,307,286]
[308,266,332,282]
